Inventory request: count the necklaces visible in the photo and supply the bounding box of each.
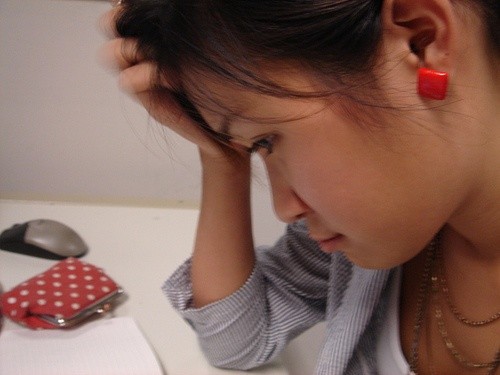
[407,229,500,375]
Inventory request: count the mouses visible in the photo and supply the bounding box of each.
[0,219,88,260]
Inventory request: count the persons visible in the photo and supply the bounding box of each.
[98,1,499,374]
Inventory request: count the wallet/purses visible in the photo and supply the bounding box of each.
[0,257,127,330]
